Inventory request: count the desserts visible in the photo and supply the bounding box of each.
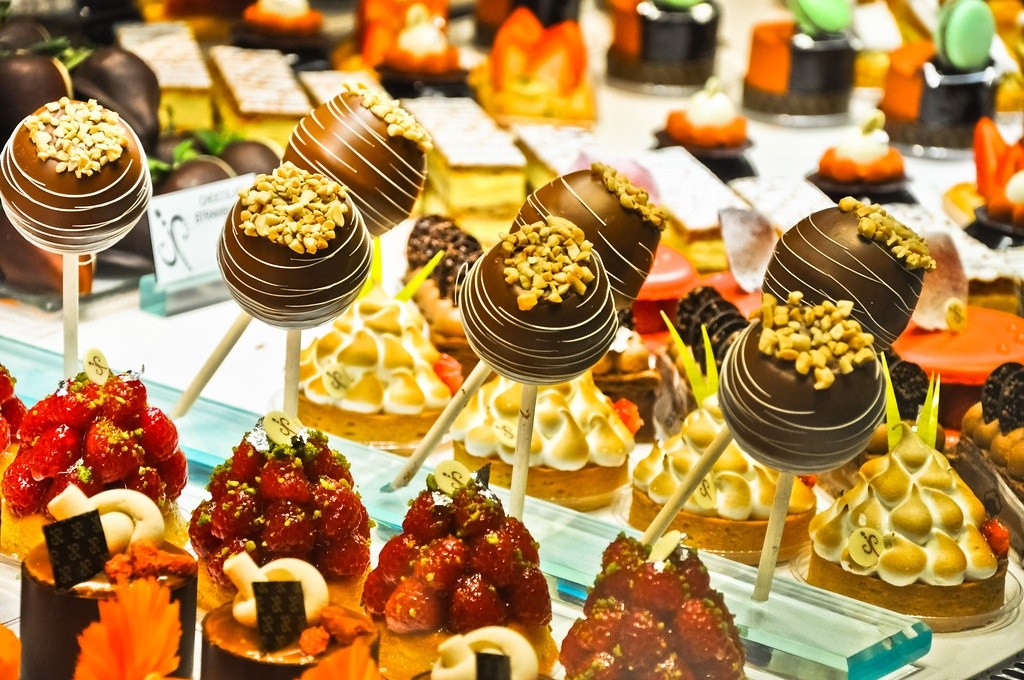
[0,0,1024,680]
